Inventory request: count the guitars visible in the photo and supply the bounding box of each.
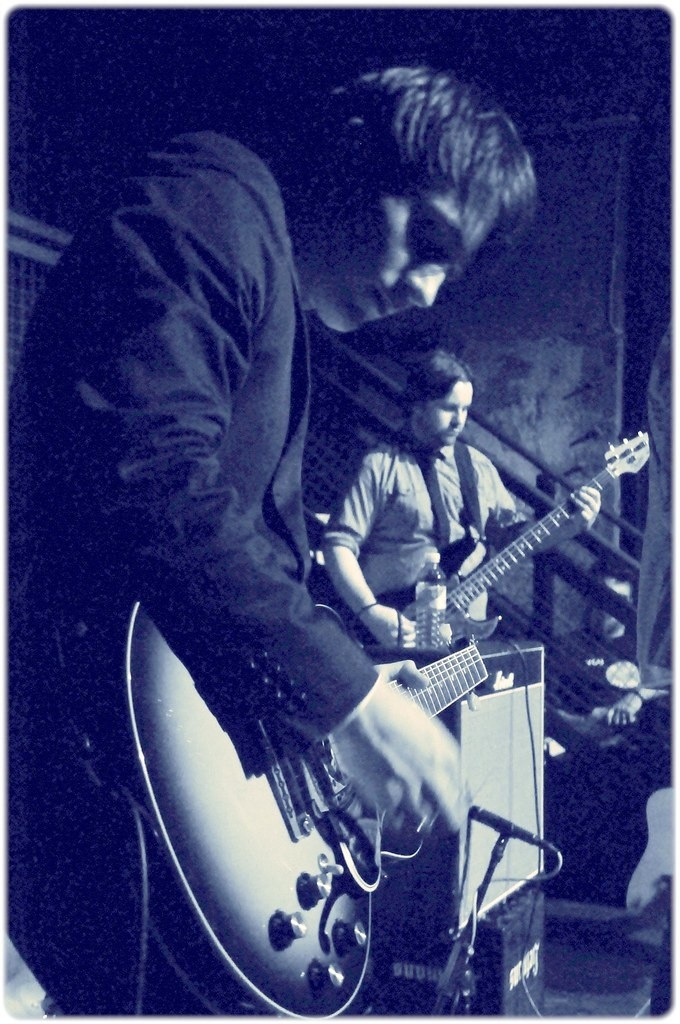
[49,594,494,1019]
[351,428,652,652]
[624,784,675,947]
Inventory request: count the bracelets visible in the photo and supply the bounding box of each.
[356,602,378,620]
[633,690,646,703]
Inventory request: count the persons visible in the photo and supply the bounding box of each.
[320,351,603,668]
[5,68,535,1017]
[602,321,674,724]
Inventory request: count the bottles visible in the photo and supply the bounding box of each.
[415,549,447,650]
